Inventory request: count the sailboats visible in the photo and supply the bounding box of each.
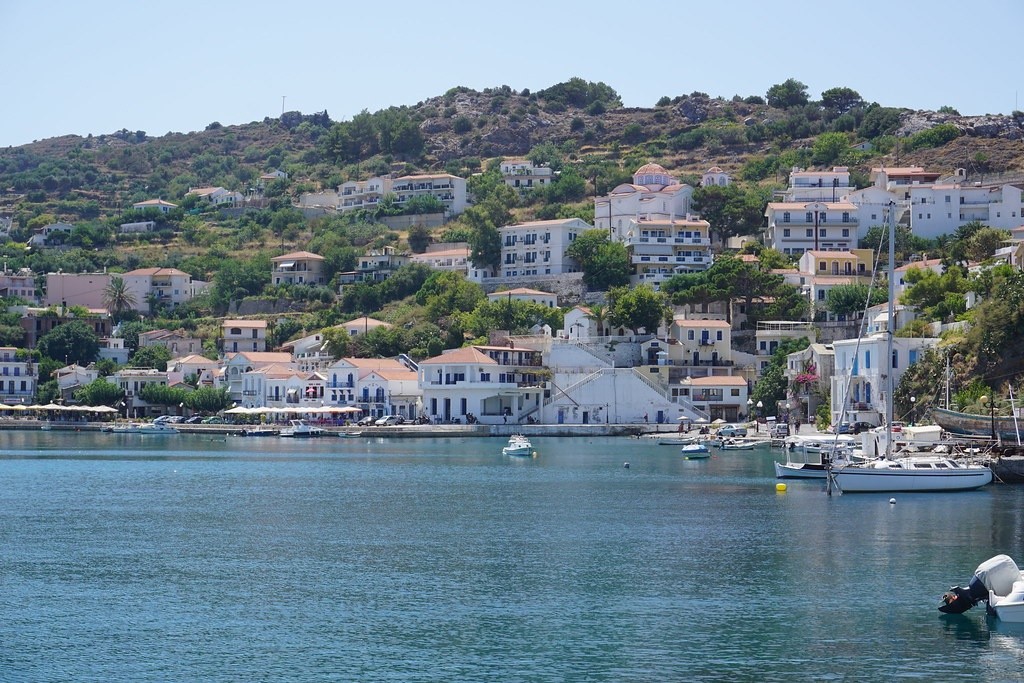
[824,201,992,493]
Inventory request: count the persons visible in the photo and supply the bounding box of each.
[854,423,860,435]
[318,416,330,426]
[776,414,782,424]
[468,413,474,424]
[688,422,691,429]
[810,414,814,427]
[644,413,648,423]
[700,426,709,434]
[780,441,782,448]
[794,421,800,434]
[727,438,735,445]
[678,421,684,431]
[304,413,310,420]
[338,414,350,426]
[718,440,724,450]
[283,416,289,426]
[527,416,537,424]
[503,412,508,424]
[783,442,785,447]
[422,412,426,419]
[783,413,787,422]
[789,442,795,452]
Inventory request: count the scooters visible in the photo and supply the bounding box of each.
[418,417,433,425]
[465,417,481,425]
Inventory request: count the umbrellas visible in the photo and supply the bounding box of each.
[224,406,362,413]
[712,418,727,428]
[676,416,688,420]
[0,403,119,413]
[696,418,708,426]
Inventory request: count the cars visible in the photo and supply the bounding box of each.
[201,416,229,424]
[718,424,747,438]
[184,417,204,424]
[848,422,876,435]
[884,421,906,432]
[162,416,187,424]
[153,415,171,425]
[770,424,787,438]
[375,416,399,427]
[357,416,379,427]
[831,421,850,435]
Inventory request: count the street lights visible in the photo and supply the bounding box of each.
[757,401,763,432]
[321,400,324,426]
[747,399,753,423]
[910,397,916,426]
[786,404,790,436]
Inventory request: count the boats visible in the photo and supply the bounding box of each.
[711,436,744,447]
[139,425,180,434]
[803,443,821,453]
[278,420,325,438]
[339,432,362,438]
[656,436,694,445]
[502,435,532,455]
[774,434,856,478]
[927,355,1024,442]
[718,438,755,450]
[240,426,279,437]
[112,422,142,433]
[938,554,1024,623]
[100,428,110,431]
[41,427,52,431]
[682,445,711,458]
